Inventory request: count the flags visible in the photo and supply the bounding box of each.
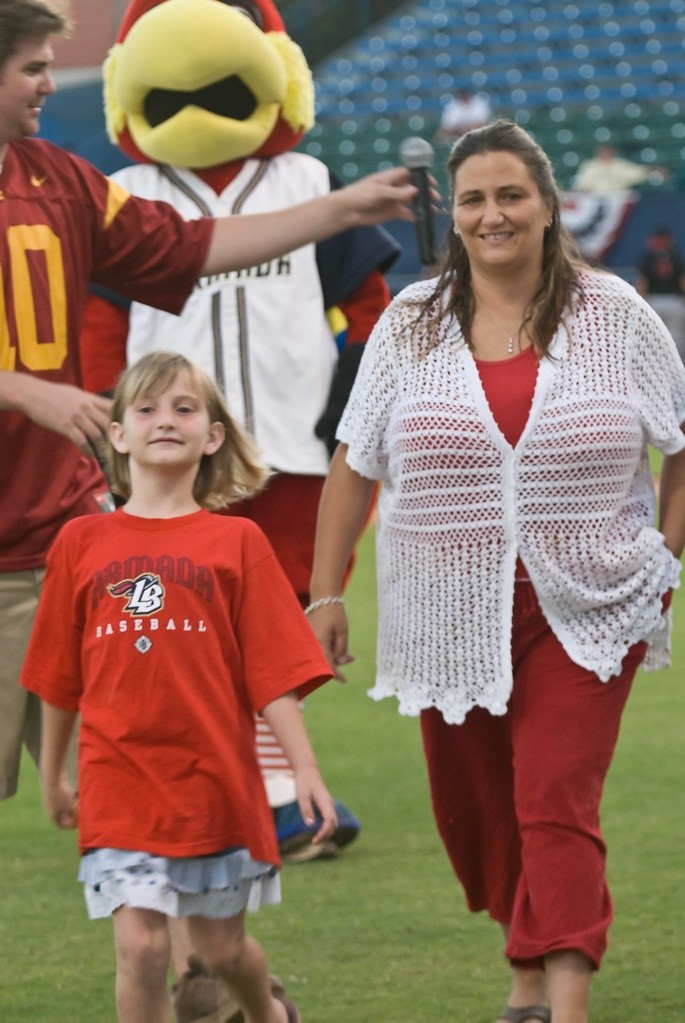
[554,188,635,260]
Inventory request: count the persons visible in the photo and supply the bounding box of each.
[634,228,685,298]
[0,0,442,800]
[310,119,685,1023]
[20,355,337,1022]
[568,143,670,190]
[434,86,491,140]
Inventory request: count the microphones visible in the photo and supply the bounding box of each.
[398,136,437,267]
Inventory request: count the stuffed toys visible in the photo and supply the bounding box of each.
[78,0,403,862]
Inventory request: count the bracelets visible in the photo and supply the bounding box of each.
[304,597,346,614]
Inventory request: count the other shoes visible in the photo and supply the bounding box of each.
[495,1003,552,1023]
[230,972,303,1023]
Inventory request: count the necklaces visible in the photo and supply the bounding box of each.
[477,305,526,354]
